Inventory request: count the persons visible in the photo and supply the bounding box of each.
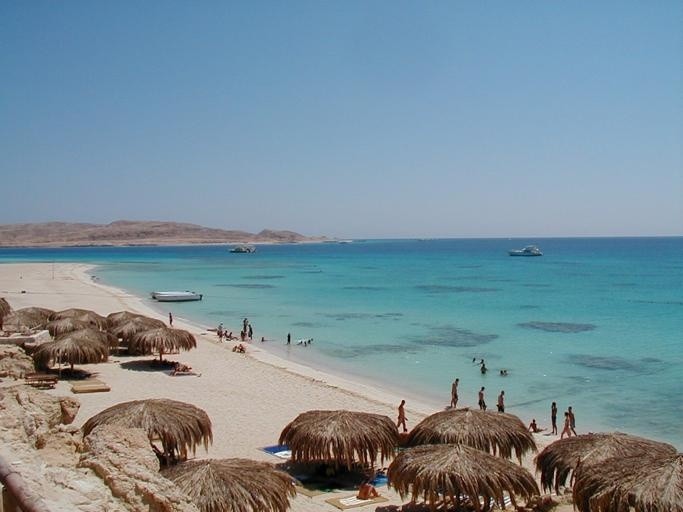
[550,402,558,435]
[451,378,459,408]
[241,318,252,341]
[528,419,548,433]
[233,344,245,353]
[496,390,504,412]
[479,387,488,410]
[175,365,192,372]
[501,370,509,375]
[168,312,173,326]
[217,322,240,341]
[356,475,381,500]
[481,364,488,374]
[396,400,408,432]
[481,360,484,363]
[561,406,577,439]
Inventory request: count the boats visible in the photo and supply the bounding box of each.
[507,245,544,257]
[150,289,203,303]
[229,244,257,254]
